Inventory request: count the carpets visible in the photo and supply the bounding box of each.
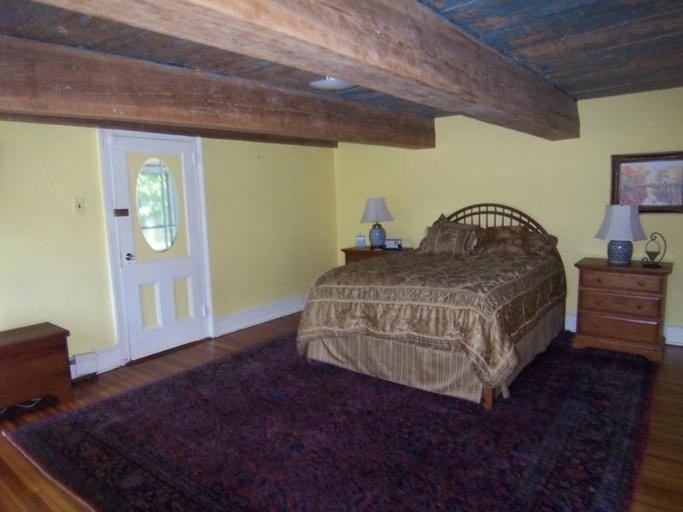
[1,326,660,512]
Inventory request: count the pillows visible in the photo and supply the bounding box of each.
[416,213,557,259]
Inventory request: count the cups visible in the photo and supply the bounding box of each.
[356,236,366,249]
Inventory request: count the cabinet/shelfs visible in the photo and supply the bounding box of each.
[0,318,74,409]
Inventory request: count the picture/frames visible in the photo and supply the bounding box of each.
[607,150,682,214]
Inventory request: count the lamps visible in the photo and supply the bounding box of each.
[358,197,396,246]
[595,203,645,265]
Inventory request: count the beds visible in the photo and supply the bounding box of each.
[294,202,568,415]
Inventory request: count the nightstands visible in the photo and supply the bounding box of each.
[568,256,674,364]
[337,246,410,263]
